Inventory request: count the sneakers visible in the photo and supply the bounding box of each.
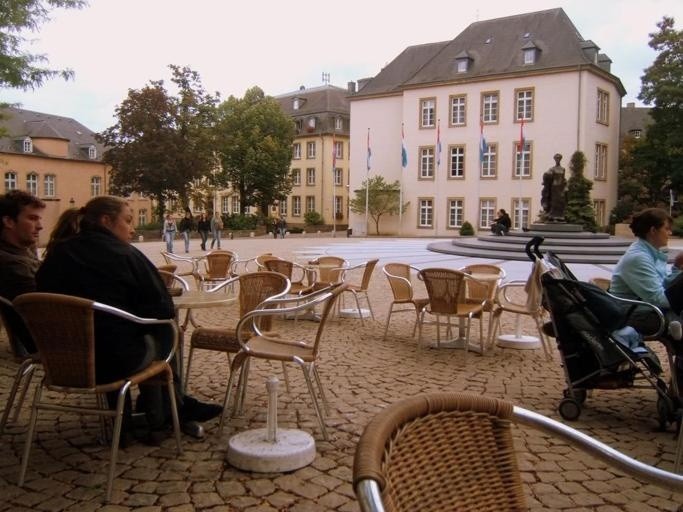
[178,395,222,426]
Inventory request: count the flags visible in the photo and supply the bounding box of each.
[519,115,525,155]
[399,119,408,169]
[435,120,442,164]
[332,130,336,168]
[478,118,487,161]
[367,128,372,171]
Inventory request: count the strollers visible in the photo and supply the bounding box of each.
[524,235,683,423]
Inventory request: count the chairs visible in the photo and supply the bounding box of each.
[351,390,682,512]
[11,293,180,502]
[0,292,41,425]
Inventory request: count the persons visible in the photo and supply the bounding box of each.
[30,195,177,437]
[0,189,222,439]
[610,204,682,313]
[271,221,277,239]
[663,309,682,342]
[277,216,287,238]
[544,153,567,222]
[159,211,224,254]
[487,209,511,235]
